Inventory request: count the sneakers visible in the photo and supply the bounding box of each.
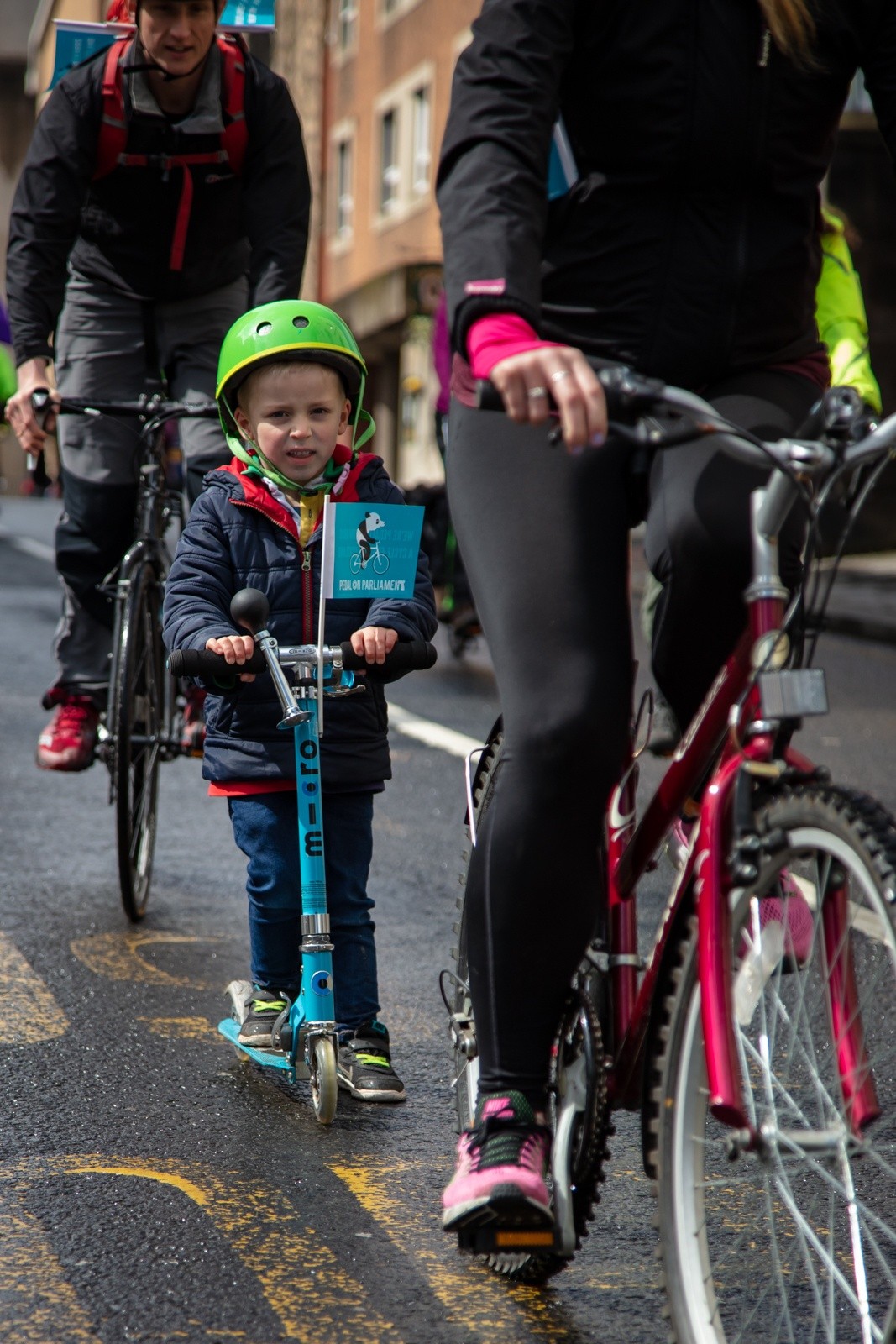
[739,866,815,973]
[334,1020,408,1105]
[440,1090,557,1231]
[182,686,207,756]
[237,985,286,1046]
[37,688,104,770]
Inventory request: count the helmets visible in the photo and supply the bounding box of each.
[215,298,367,431]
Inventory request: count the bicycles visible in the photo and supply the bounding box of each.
[478,356,896,1344]
[24,387,223,922]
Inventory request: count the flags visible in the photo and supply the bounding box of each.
[327,498,425,603]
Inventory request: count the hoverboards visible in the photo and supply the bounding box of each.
[171,590,436,1125]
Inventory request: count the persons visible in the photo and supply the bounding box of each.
[643,175,884,754]
[4,0,311,774]
[436,0,896,1236]
[163,299,438,1103]
[432,292,458,623]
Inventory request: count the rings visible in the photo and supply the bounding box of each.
[526,386,548,398]
